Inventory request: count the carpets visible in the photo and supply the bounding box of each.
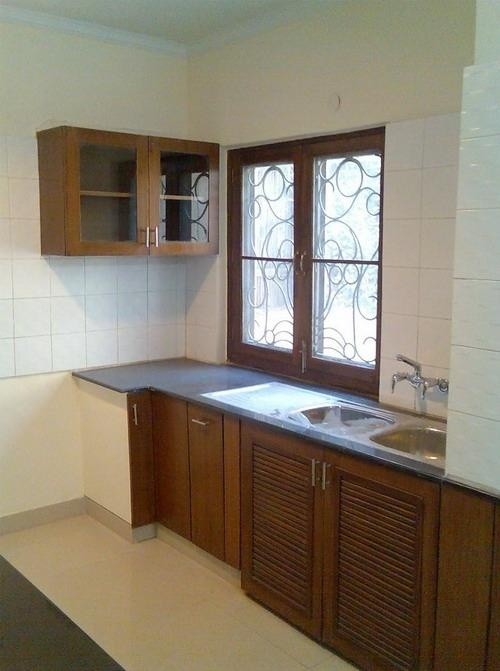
[0,556,127,671]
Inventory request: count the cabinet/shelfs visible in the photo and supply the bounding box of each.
[434,481,500,670]
[128,391,241,592]
[242,418,440,670]
[35,125,219,256]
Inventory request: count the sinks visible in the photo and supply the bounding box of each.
[273,398,396,441]
[366,417,446,466]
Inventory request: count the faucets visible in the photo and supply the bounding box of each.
[388,352,441,402]
